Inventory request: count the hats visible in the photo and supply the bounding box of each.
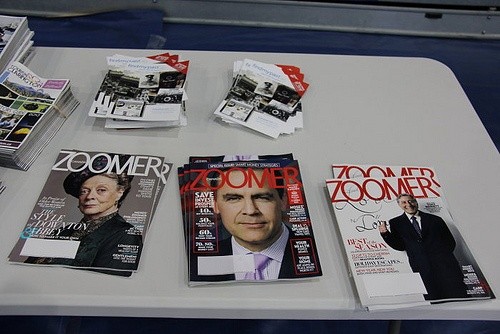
[63,153,134,199]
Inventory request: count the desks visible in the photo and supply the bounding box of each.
[0,43,500,334]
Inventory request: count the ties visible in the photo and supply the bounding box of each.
[244,254,271,280]
[411,216,423,240]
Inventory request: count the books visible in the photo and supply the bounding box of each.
[177,152,323,288]
[212,58,310,138]
[325,162,497,311]
[7,149,175,278]
[88,51,193,130]
[0,13,37,76]
[0,61,81,172]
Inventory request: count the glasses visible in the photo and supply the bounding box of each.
[399,200,416,206]
